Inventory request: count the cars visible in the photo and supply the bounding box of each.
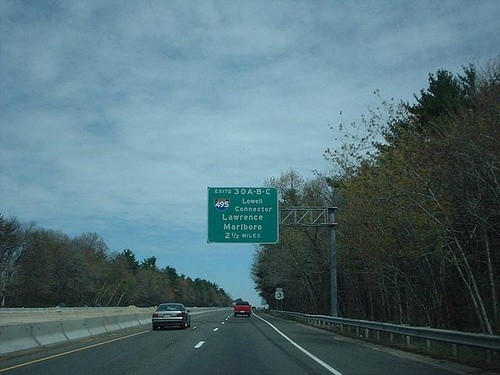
[152,303,191,330]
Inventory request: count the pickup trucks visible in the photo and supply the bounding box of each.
[234,301,252,317]
